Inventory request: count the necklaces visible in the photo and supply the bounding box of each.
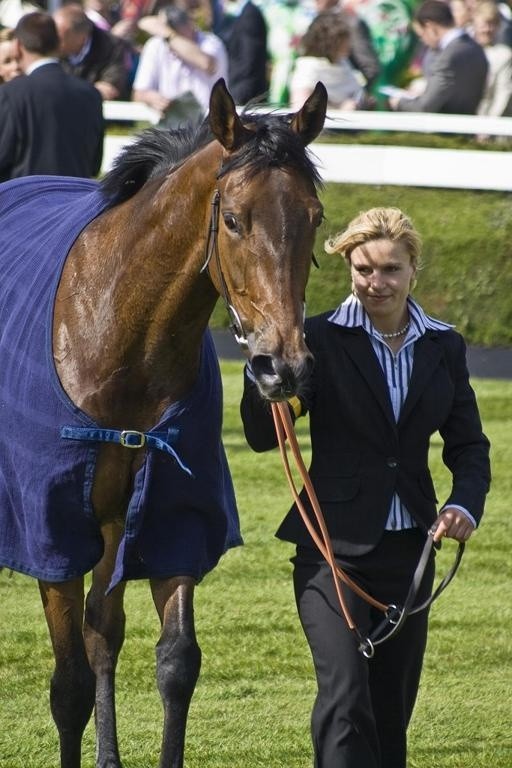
[379,322,410,339]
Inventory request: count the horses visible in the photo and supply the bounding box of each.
[0,76,350,768]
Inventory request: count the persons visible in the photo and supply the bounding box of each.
[240,207,491,768]
[2,14,106,183]
[0,0,512,148]
